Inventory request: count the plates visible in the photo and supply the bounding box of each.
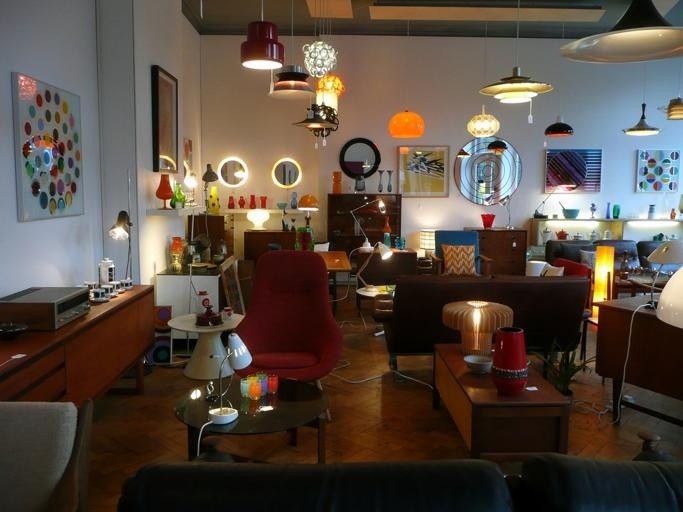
[189,263,210,267]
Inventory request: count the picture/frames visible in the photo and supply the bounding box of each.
[150,60,179,173]
[395,145,449,198]
[217,257,244,315]
[13,72,86,222]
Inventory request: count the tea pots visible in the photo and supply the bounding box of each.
[539,229,584,243]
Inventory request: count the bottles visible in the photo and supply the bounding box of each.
[362,237,370,248]
[227,193,256,208]
[619,249,628,280]
[192,253,200,263]
[670,208,675,220]
[168,236,184,273]
[387,170,393,192]
[222,307,234,320]
[172,183,185,208]
[216,238,227,256]
[196,290,212,316]
[648,204,656,221]
[97,257,114,285]
[290,191,297,209]
[588,228,610,241]
[378,170,383,192]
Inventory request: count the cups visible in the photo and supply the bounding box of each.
[394,236,406,250]
[481,214,495,230]
[90,280,133,304]
[332,171,341,183]
[383,233,390,248]
[259,196,267,208]
[612,204,621,219]
[239,370,278,401]
[332,183,341,193]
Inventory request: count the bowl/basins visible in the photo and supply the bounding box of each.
[463,355,494,375]
[212,254,225,263]
[276,202,287,209]
[417,259,432,266]
[562,209,580,219]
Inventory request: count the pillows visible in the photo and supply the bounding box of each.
[561,243,597,268]
[435,231,481,276]
[547,240,682,275]
[540,259,590,274]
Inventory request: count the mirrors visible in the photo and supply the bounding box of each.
[271,157,302,189]
[215,154,247,189]
[338,136,378,179]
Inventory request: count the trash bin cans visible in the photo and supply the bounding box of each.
[121,343,152,379]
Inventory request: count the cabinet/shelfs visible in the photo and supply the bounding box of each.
[188,213,234,259]
[326,192,402,252]
[0,283,158,422]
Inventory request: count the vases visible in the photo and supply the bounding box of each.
[167,235,184,261]
[169,254,182,272]
[605,202,658,221]
[155,170,400,210]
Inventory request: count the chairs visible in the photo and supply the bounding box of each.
[226,249,345,379]
[1,396,91,511]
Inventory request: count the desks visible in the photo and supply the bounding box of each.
[463,227,527,275]
[155,257,231,340]
[240,227,313,254]
[615,271,670,299]
[315,249,352,317]
[424,345,571,460]
[357,247,417,285]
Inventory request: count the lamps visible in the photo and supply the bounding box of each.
[487,1,507,150]
[532,182,577,219]
[543,1,574,137]
[296,192,322,227]
[497,195,515,228]
[420,230,435,260]
[442,301,514,355]
[108,209,133,283]
[244,209,270,232]
[667,58,683,121]
[207,332,252,426]
[388,4,425,138]
[657,264,683,329]
[348,197,390,244]
[201,162,218,239]
[268,2,345,150]
[241,1,284,70]
[478,0,555,124]
[355,242,394,291]
[599,240,683,423]
[559,2,683,64]
[621,61,662,137]
[466,1,500,138]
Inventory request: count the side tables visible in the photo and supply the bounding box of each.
[166,311,245,381]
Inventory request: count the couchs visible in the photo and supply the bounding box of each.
[382,276,591,369]
[114,461,683,512]
